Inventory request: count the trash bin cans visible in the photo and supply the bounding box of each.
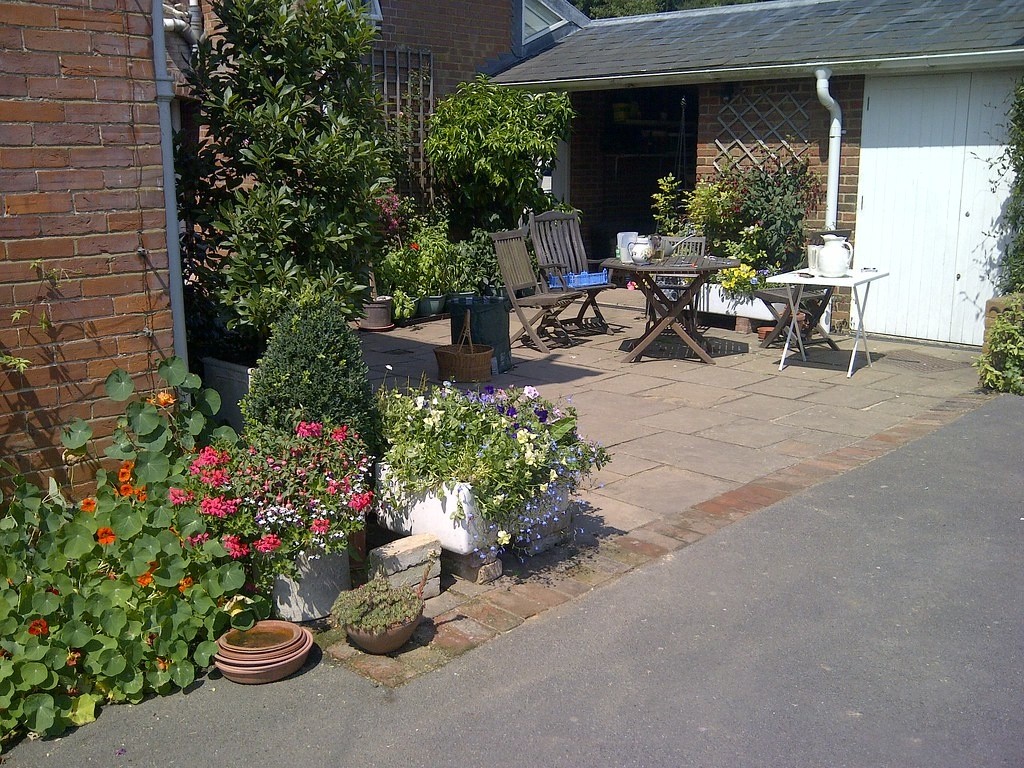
[447,295,512,375]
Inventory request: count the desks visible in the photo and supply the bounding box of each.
[766,267,890,378]
[601,254,742,365]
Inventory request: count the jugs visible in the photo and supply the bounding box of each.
[627,235,657,265]
[617,232,638,263]
[817,234,853,278]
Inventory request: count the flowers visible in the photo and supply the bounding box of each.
[717,256,783,304]
[0,353,615,741]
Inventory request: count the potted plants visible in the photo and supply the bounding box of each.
[355,221,511,331]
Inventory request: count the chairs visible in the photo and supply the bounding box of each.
[521,210,618,347]
[491,229,583,354]
[646,236,706,335]
